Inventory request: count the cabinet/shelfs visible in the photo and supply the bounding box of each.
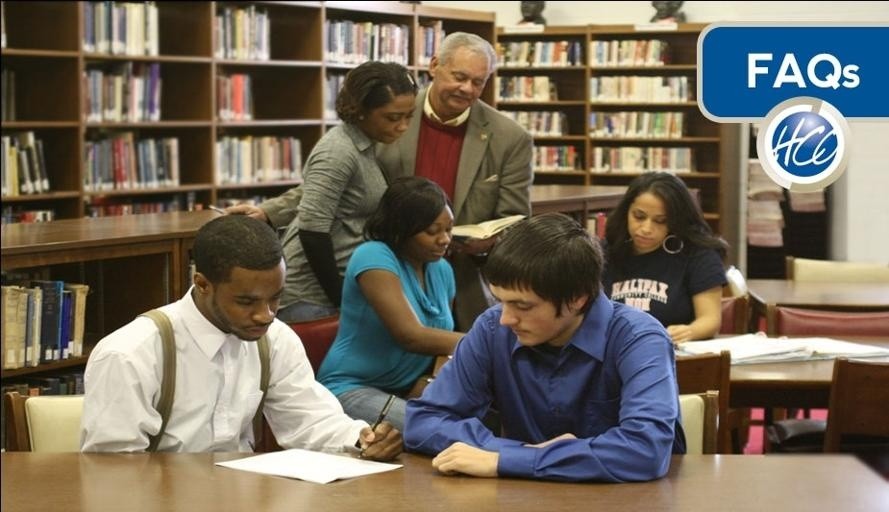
[1,0,752,450]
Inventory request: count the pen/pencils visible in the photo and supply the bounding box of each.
[208,205,224,214]
[357,395,396,459]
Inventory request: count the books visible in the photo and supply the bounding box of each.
[0,2,302,222]
[1,267,90,451]
[493,34,697,173]
[586,211,597,242]
[673,328,888,367]
[451,213,529,250]
[321,17,445,121]
[592,209,606,242]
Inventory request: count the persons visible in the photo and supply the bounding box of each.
[77,214,405,462]
[267,60,419,326]
[314,174,471,431]
[402,209,688,485]
[604,169,729,343]
[221,31,538,333]
[518,1,545,26]
[651,1,687,23]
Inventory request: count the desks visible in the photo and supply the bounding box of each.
[745,279,885,334]
[2,450,888,511]
[674,335,885,453]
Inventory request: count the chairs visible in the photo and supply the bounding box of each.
[666,255,889,454]
